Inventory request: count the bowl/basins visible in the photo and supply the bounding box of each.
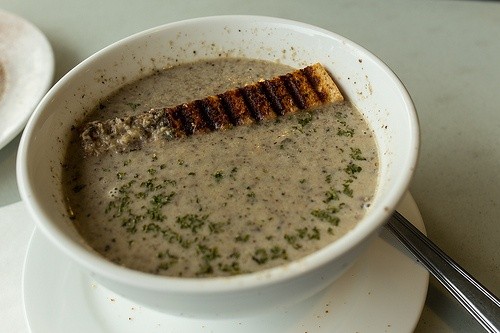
[13,16,418,321]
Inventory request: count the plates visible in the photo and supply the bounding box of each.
[22,190,428,333]
[0,9,56,150]
